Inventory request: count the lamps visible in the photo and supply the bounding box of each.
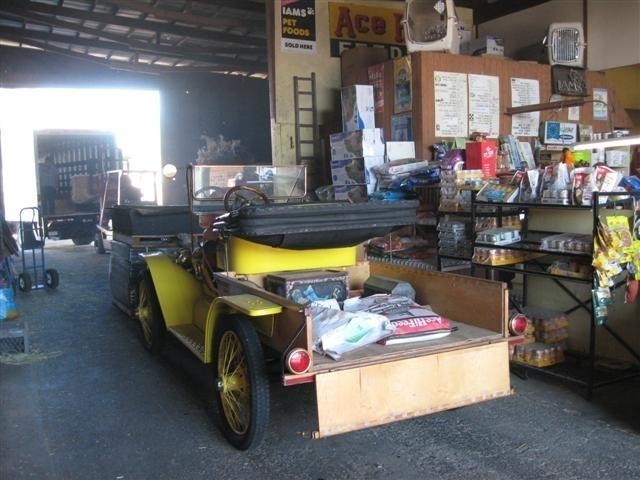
[505,98,640,152]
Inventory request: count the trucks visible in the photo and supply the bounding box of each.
[32,129,143,247]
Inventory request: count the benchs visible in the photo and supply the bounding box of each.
[213,198,420,276]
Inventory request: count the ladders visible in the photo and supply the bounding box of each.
[292,71,321,195]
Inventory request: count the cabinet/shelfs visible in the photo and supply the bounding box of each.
[435,187,640,401]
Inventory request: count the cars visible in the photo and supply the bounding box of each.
[136,165,515,450]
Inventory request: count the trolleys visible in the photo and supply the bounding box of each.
[18,207,58,292]
